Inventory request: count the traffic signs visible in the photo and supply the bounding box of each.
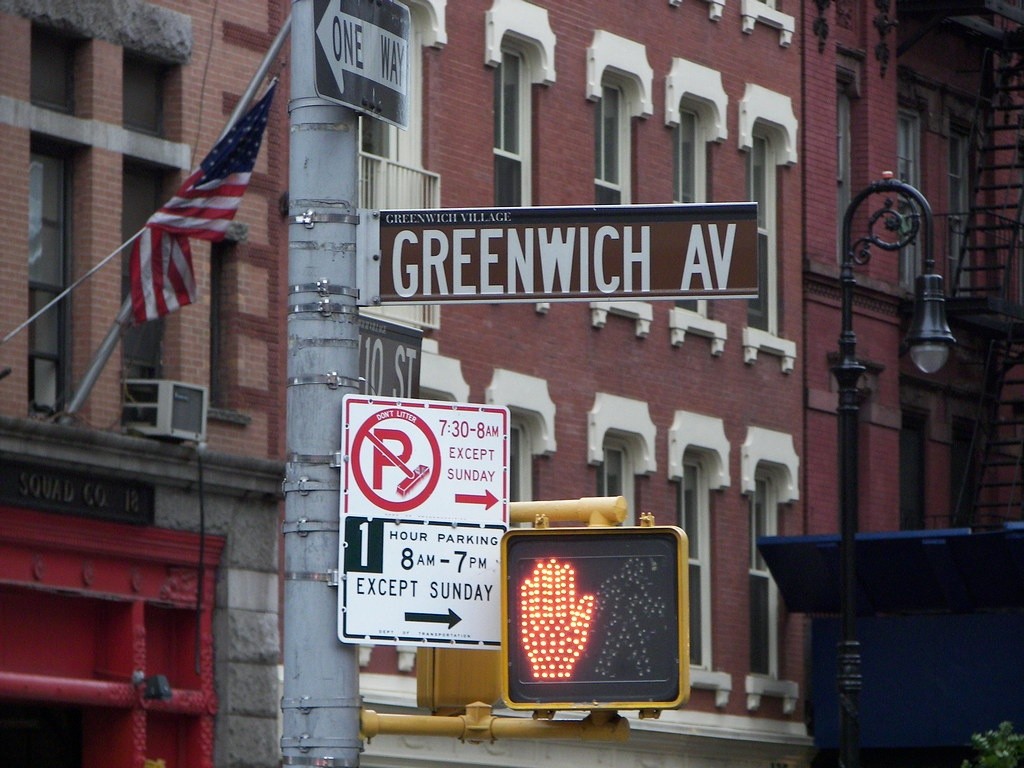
[338,515,501,653]
[309,0,413,131]
[339,392,512,529]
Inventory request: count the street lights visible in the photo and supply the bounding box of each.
[831,167,957,768]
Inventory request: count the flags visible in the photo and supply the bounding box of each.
[130,83,277,325]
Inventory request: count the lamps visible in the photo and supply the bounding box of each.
[142,674,172,700]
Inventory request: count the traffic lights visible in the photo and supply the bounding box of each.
[500,524,691,713]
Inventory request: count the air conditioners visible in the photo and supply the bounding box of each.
[120,378,209,442]
